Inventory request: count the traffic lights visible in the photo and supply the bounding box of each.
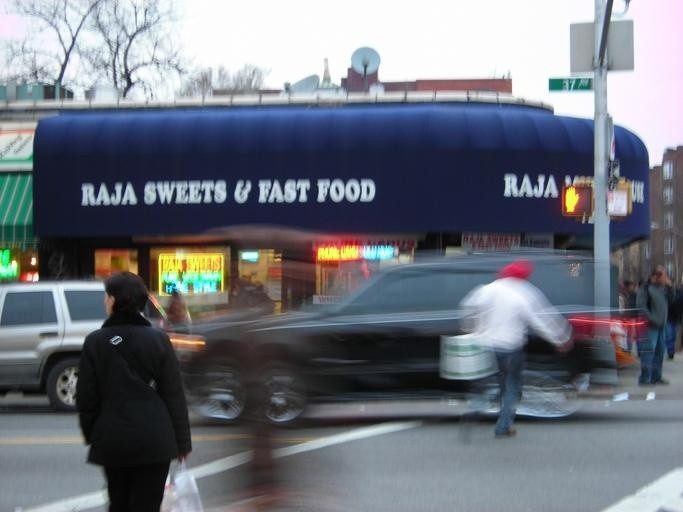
[560,183,593,219]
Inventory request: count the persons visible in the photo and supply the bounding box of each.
[636,265,676,385]
[76,271,191,512]
[618,276,683,360]
[167,290,191,324]
[457,260,574,438]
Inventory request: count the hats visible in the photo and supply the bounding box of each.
[501,260,534,278]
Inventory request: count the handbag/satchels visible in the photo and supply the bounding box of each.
[160,458,203,511]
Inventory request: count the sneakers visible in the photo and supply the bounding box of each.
[638,377,668,388]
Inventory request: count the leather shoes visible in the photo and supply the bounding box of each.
[495,428,517,438]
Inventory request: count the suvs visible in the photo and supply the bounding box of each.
[2,276,170,410]
[178,247,604,431]
